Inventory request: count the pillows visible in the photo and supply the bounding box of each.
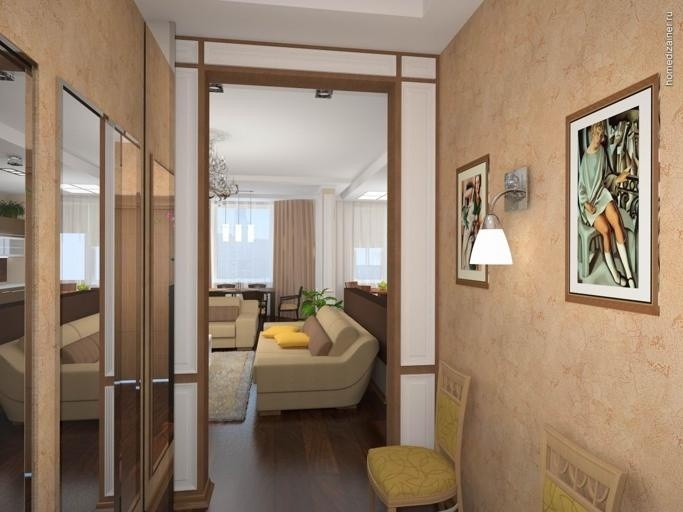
[17,334,25,353]
[209,306,239,322]
[61,312,100,364]
[260,304,359,357]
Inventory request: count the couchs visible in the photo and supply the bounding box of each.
[0,338,24,431]
[251,304,381,418]
[209,300,259,351]
[60,312,101,421]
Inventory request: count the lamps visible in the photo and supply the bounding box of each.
[209,128,238,207]
[221,189,256,243]
[468,165,531,265]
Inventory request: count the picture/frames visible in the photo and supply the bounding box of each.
[456,154,490,289]
[565,72,660,317]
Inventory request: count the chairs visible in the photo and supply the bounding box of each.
[357,286,370,293]
[209,283,303,322]
[539,422,628,512]
[367,360,472,512]
[345,281,358,289]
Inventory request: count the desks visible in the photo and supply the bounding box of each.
[371,288,387,296]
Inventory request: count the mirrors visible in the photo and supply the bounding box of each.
[104,115,144,512]
[0,32,38,512]
[60,78,101,512]
[149,153,175,478]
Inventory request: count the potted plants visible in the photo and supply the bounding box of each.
[0,199,24,218]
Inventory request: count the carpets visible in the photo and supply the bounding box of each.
[208,350,255,422]
